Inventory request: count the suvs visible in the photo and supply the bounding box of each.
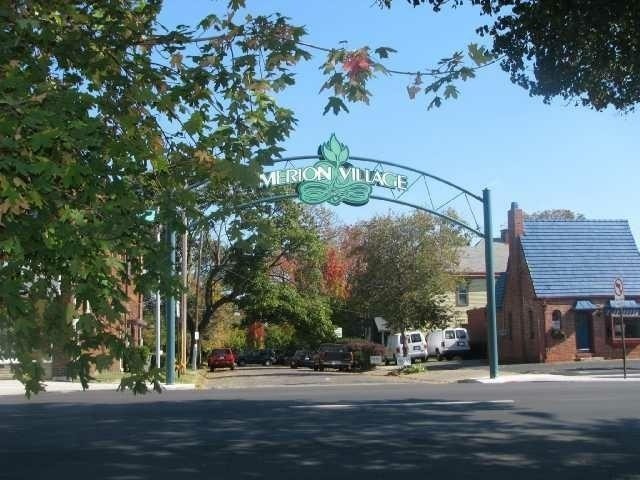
[206,347,236,371]
[236,348,279,366]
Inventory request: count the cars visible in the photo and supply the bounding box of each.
[288,349,314,369]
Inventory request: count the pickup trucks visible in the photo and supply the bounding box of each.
[312,342,355,373]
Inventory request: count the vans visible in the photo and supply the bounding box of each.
[423,327,472,361]
[380,328,429,366]
